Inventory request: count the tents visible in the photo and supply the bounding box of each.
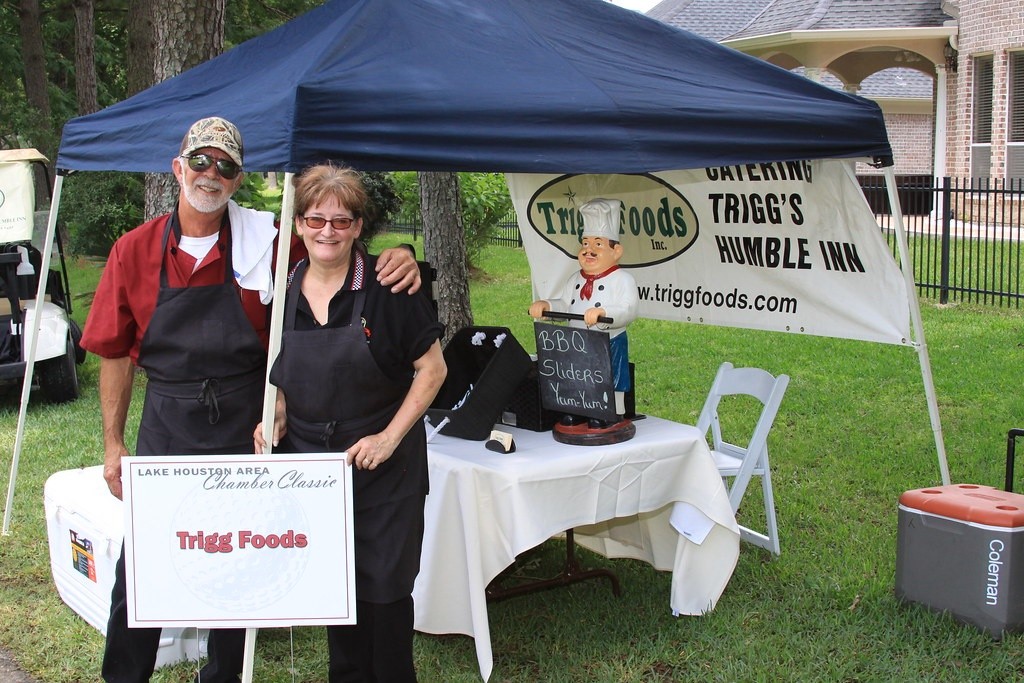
[1,1,951,683]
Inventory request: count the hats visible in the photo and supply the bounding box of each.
[179,115,244,167]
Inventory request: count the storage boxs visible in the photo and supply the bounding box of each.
[896,482,1023,641]
[426,325,636,441]
[45,469,212,667]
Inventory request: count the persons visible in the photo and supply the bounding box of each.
[529,198,640,429]
[253,165,448,683]
[79,117,422,682]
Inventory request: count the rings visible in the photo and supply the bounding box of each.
[372,462,378,467]
[365,458,371,463]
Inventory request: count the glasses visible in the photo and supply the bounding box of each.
[301,215,356,230]
[179,153,243,180]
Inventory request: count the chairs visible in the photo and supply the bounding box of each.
[692,362,791,558]
[0,251,24,317]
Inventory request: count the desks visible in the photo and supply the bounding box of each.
[414,414,704,610]
